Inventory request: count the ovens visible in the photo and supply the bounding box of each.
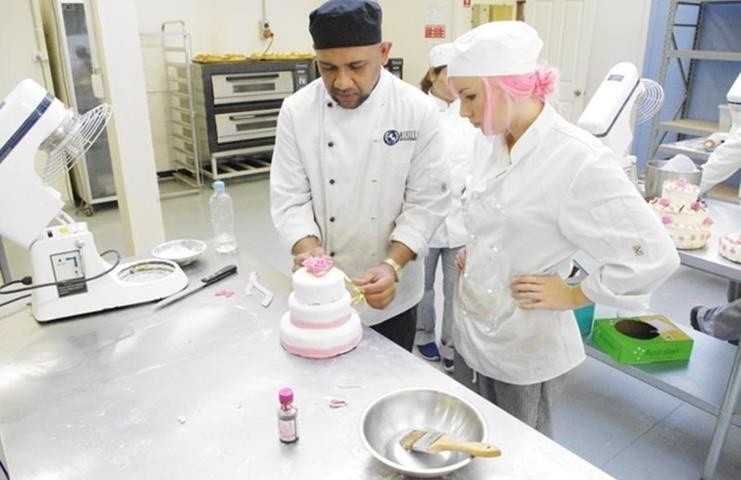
[190,61,316,157]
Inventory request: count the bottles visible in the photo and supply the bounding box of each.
[208,178,237,254]
[276,387,300,445]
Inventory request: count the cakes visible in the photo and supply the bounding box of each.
[718,231,741,265]
[277,254,363,359]
[649,176,714,250]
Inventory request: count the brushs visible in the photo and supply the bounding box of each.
[397,426,502,460]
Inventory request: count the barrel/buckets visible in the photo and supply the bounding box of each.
[644,159,703,199]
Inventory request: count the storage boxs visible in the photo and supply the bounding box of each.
[573,304,694,367]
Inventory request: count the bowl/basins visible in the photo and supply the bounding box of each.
[359,386,486,478]
[151,238,209,266]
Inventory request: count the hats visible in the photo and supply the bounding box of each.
[309,0,381,49]
[427,20,544,78]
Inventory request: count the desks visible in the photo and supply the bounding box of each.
[0,236,619,479]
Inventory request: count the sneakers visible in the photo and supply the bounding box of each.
[416,341,455,373]
[688,304,718,338]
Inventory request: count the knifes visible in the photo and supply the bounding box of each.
[150,264,237,308]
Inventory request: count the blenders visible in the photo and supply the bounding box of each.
[578,61,705,199]
[0,79,190,323]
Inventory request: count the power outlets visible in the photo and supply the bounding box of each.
[259,20,274,40]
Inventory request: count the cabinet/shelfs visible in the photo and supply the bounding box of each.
[580,168,741,479]
[646,0,740,206]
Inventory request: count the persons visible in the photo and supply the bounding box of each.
[446,19,682,443]
[415,39,480,374]
[269,0,451,353]
[690,115,741,202]
[691,279,741,346]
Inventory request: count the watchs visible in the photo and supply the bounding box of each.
[382,256,403,283]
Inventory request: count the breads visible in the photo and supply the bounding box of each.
[193,52,314,64]
